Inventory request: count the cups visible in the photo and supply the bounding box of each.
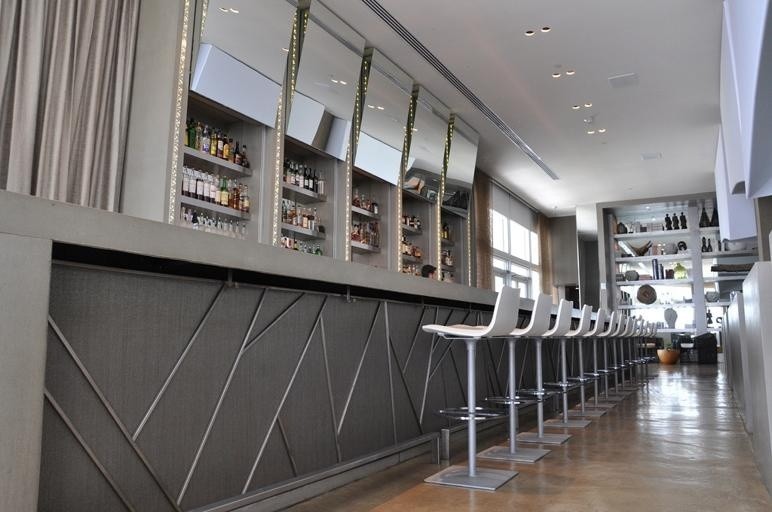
[652,259,664,280]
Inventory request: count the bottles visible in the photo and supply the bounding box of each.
[701,236,712,252]
[185,118,252,169]
[281,157,328,257]
[181,166,249,241]
[350,186,380,250]
[402,210,424,278]
[439,218,456,281]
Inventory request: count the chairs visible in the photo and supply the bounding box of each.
[422,285,659,491]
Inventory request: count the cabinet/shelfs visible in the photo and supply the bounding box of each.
[598,200,767,358]
[178,95,472,285]
